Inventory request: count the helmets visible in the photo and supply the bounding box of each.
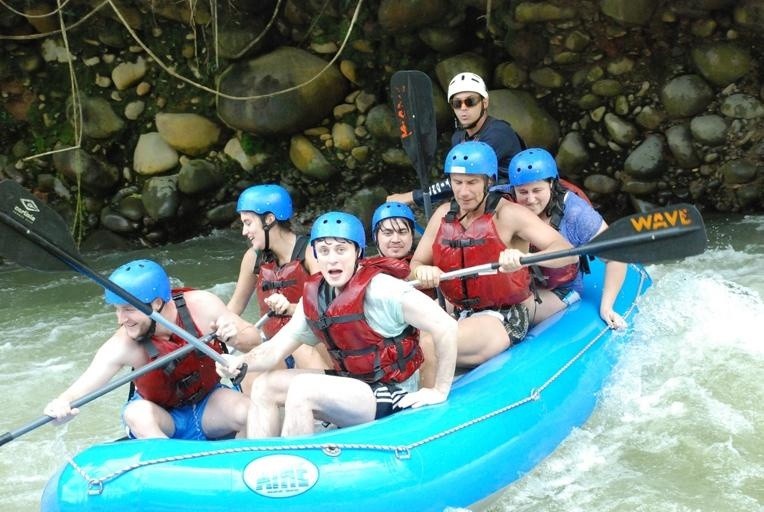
[443,141,498,185]
[507,147,559,188]
[446,72,488,105]
[235,184,293,222]
[370,201,416,241]
[103,258,171,306]
[309,210,365,260]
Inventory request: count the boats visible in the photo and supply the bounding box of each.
[42,258,653,511]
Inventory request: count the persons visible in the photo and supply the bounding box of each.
[213,211,462,439]
[221,184,335,366]
[41,256,261,439]
[368,202,440,299]
[493,152,631,333]
[385,73,527,203]
[406,142,579,388]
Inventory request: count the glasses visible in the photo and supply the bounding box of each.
[449,95,482,110]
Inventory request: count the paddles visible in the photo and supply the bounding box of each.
[407,204,707,288]
[389,71,438,223]
[0,179,247,386]
